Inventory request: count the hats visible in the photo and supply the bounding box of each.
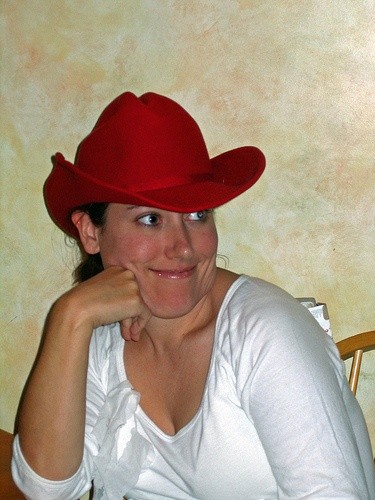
[43,90,267,240]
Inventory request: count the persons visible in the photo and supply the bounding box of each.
[10,91,374,500]
